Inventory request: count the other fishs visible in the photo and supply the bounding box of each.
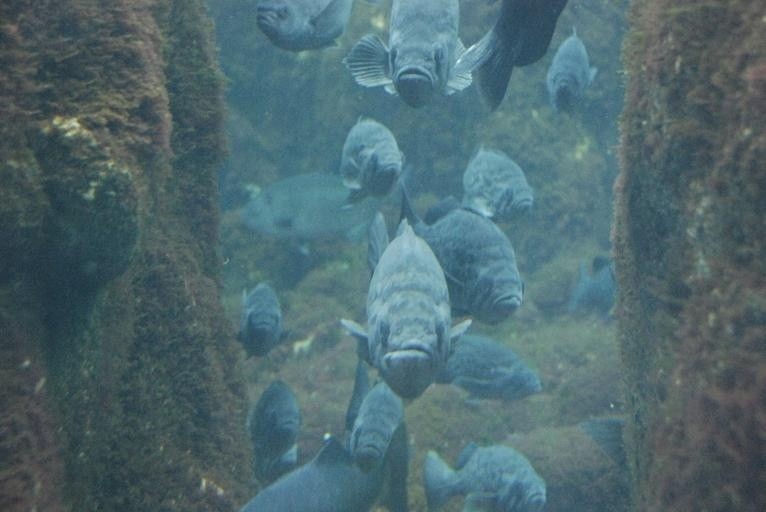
[233,0,626,511]
